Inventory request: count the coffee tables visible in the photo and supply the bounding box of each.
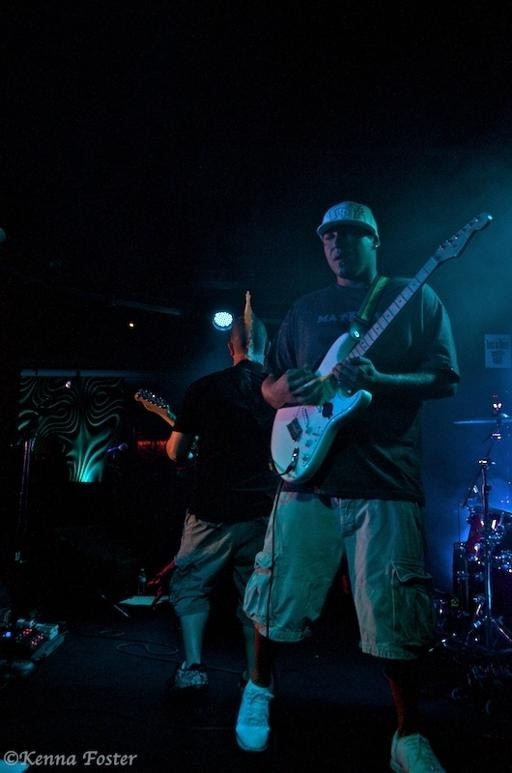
[452,417,512,429]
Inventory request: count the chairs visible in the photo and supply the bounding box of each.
[236,676,275,753]
[389,729,446,773]
[169,663,209,691]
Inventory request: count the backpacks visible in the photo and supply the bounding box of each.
[467,624,510,689]
[464,506,512,568]
[454,543,470,600]
[438,600,472,637]
[470,554,512,628]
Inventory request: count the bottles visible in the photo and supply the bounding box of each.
[316,201,380,240]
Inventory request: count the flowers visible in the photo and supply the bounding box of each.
[99,443,129,459]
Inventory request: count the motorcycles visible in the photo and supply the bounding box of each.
[461,414,510,650]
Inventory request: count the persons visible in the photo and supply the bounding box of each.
[159,312,270,687]
[237,195,459,773]
